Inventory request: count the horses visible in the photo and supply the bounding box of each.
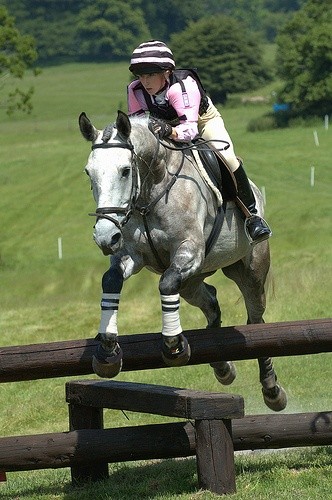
[78,109,288,413]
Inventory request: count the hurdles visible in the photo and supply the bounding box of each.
[0,317,332,496]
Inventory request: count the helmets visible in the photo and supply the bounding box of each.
[129,40,176,73]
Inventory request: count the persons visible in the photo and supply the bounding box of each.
[126,41,273,244]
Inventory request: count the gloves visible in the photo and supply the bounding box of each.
[150,120,173,140]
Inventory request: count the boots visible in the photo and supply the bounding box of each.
[233,164,270,240]
